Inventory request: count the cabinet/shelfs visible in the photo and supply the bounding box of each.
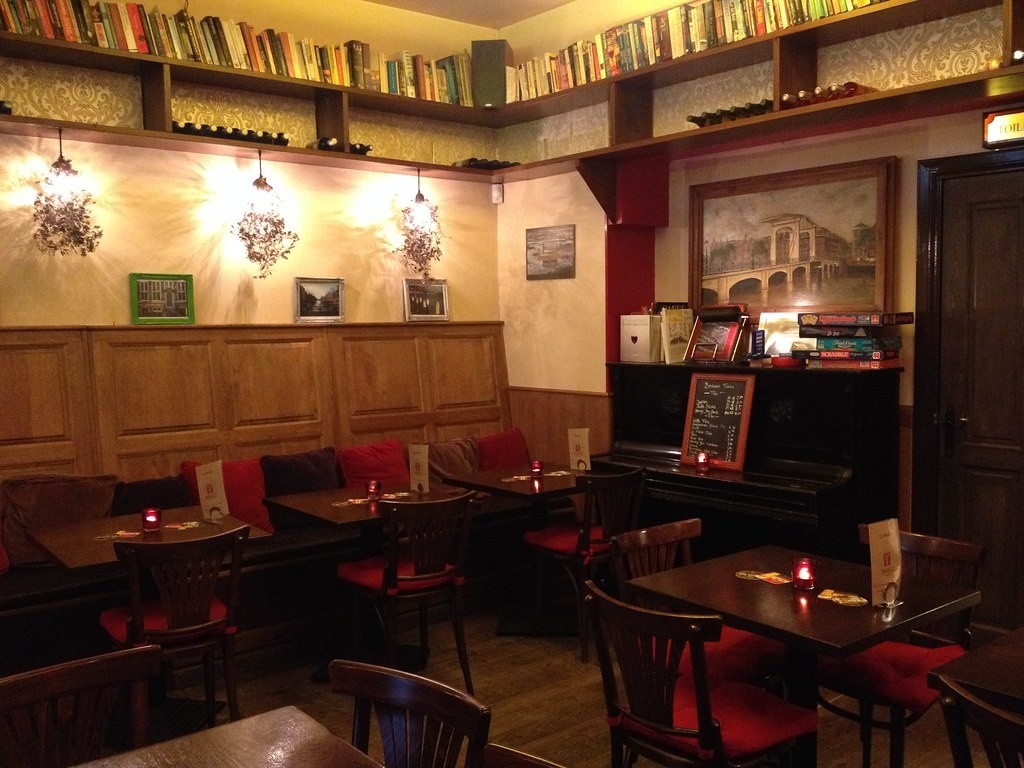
[1,1,1024,184]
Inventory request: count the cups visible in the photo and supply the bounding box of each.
[366,480,381,500]
[142,507,161,532]
[790,557,822,590]
[528,460,544,478]
[694,451,709,473]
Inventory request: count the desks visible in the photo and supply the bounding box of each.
[263,486,461,672]
[71,706,385,768]
[29,504,251,729]
[442,472,581,636]
[626,542,980,768]
[927,623,1023,712]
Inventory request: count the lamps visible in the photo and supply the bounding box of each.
[31,127,103,256]
[229,146,299,279]
[391,165,449,280]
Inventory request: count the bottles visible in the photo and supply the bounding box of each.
[687,98,773,127]
[172,121,289,146]
[450,157,521,171]
[782,82,879,108]
[350,143,360,152]
[0,100,13,114]
[305,137,338,151]
[353,143,373,155]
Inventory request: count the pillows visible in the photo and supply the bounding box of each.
[0,428,530,567]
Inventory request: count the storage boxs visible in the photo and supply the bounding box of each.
[618,315,662,363]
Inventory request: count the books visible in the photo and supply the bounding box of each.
[618,309,749,364]
[792,309,914,371]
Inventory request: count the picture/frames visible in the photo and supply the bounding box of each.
[403,277,449,323]
[751,313,817,357]
[130,272,195,327]
[686,153,901,352]
[294,277,346,326]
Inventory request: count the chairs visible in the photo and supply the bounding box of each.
[936,671,1023,768]
[485,743,564,768]
[0,462,988,768]
